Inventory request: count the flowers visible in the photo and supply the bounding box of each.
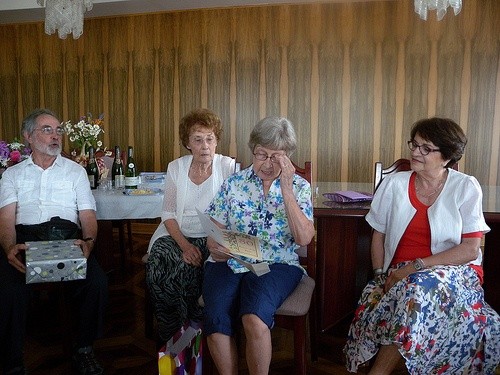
[0,138,31,168]
[60,113,114,178]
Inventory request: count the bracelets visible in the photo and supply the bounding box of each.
[372,268,383,273]
[83,237,95,248]
[412,258,425,271]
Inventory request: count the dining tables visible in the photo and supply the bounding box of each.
[91,173,173,276]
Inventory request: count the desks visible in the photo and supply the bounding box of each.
[314,183,500,326]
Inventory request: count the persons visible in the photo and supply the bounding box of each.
[145,107,241,346]
[204,115,316,375]
[0,109,108,375]
[343,117,500,375]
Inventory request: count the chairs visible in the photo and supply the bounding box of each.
[374,158,458,194]
[72,149,133,279]
[200,159,317,375]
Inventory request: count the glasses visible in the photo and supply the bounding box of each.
[408,140,441,156]
[252,153,280,163]
[29,127,65,136]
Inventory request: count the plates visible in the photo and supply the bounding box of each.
[124,188,165,196]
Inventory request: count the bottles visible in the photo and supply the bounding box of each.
[125,146,138,190]
[85,145,99,189]
[112,146,125,188]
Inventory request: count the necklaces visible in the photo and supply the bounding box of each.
[416,169,448,197]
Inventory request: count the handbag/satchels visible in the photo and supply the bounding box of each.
[158,319,202,375]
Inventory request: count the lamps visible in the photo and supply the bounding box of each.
[37,0,93,39]
[414,0,463,22]
